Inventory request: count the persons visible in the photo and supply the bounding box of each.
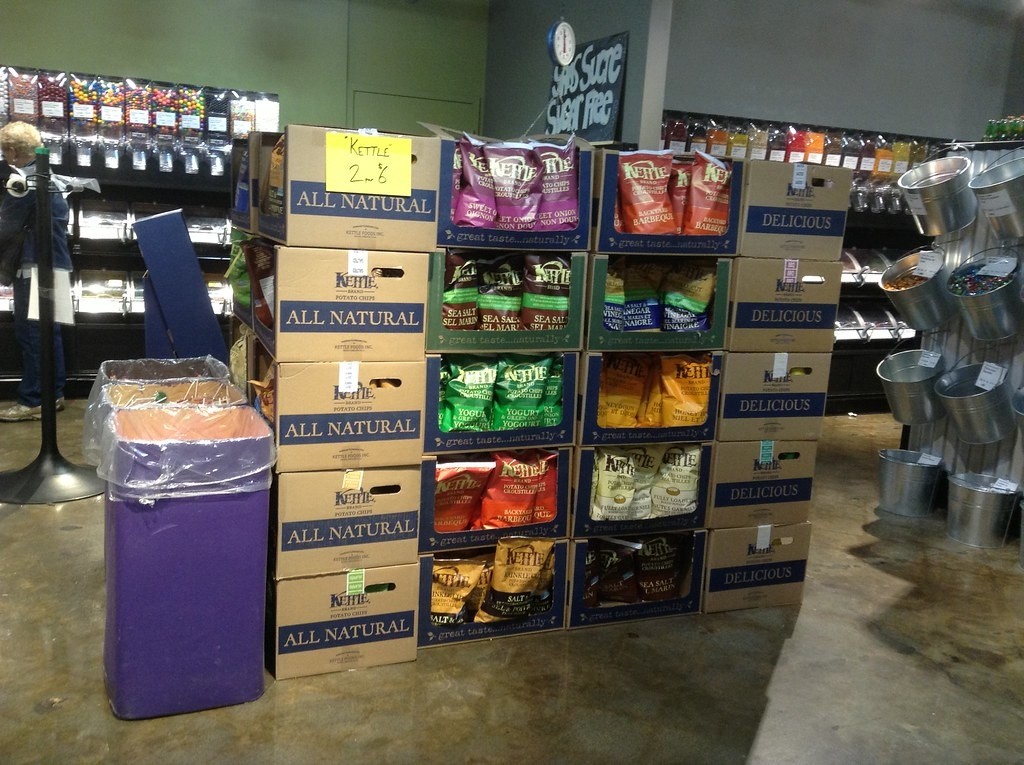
[0,120,73,422]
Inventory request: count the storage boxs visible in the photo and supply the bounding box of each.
[230,121,855,682]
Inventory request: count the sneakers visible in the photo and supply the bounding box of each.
[0,402,42,422]
[54,396,65,411]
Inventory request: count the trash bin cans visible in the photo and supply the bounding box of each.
[100,356,248,406]
[104,405,275,720]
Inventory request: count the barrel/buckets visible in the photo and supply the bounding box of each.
[945,473,1020,549]
[877,245,963,331]
[933,348,1018,445]
[896,145,978,236]
[875,335,947,425]
[944,246,1024,341]
[878,449,943,518]
[968,146,1024,240]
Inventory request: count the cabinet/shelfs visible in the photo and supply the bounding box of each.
[657,109,979,415]
[0,65,279,399]
